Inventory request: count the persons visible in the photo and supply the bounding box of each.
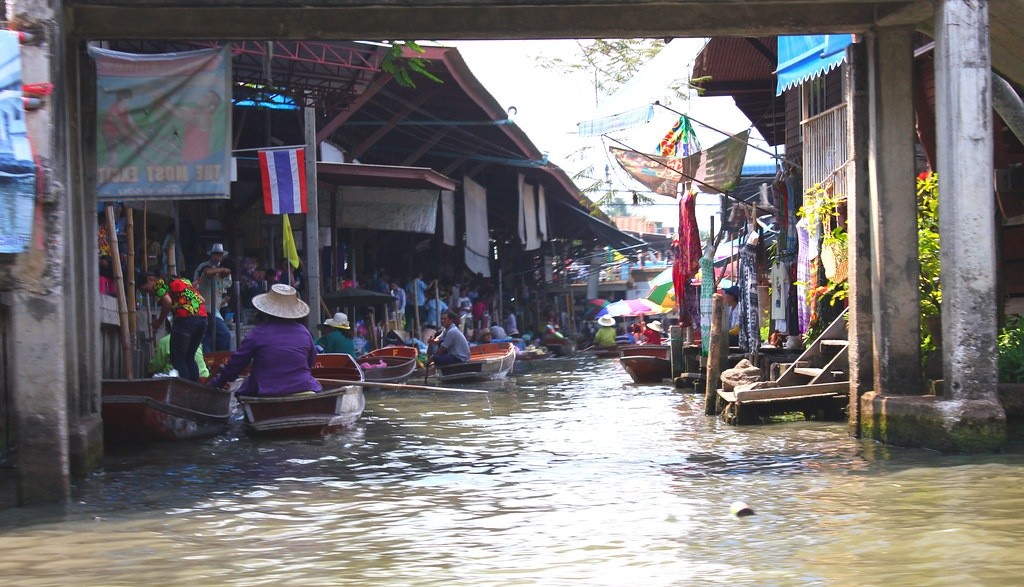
[137,268,208,382]
[198,263,232,352]
[145,319,210,379]
[678,180,703,278]
[194,243,229,353]
[722,286,742,345]
[104,89,221,166]
[314,271,669,366]
[207,284,322,396]
[738,224,762,353]
[698,244,715,356]
[113,202,186,275]
[239,250,305,325]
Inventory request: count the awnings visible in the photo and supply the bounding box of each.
[553,197,658,283]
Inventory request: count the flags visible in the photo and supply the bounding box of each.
[259,147,307,216]
[282,214,299,269]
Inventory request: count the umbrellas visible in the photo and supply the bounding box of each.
[323,288,399,336]
[584,299,611,310]
[646,267,678,310]
[639,298,672,328]
[595,299,652,334]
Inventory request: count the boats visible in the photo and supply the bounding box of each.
[234,353,367,441]
[516,340,579,360]
[354,345,418,388]
[619,355,670,385]
[593,336,671,359]
[435,341,516,383]
[98,350,250,445]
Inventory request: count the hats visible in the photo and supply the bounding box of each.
[598,315,615,326]
[647,320,664,332]
[509,328,520,338]
[478,328,491,340]
[553,324,560,328]
[206,243,228,256]
[251,284,310,318]
[722,286,739,297]
[323,313,350,329]
